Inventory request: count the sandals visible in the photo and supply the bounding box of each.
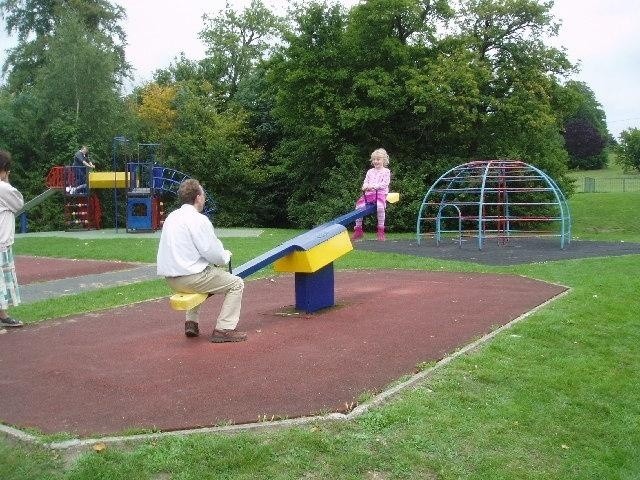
[0,317,23,327]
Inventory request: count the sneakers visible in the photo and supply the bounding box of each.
[351,227,364,240]
[211,329,246,343]
[377,227,385,241]
[185,320,199,337]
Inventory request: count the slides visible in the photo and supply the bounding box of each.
[12,188,63,219]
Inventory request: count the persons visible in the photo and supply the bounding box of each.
[73,145,95,194]
[350,148,390,241]
[157,179,248,343]
[0,150,24,326]
